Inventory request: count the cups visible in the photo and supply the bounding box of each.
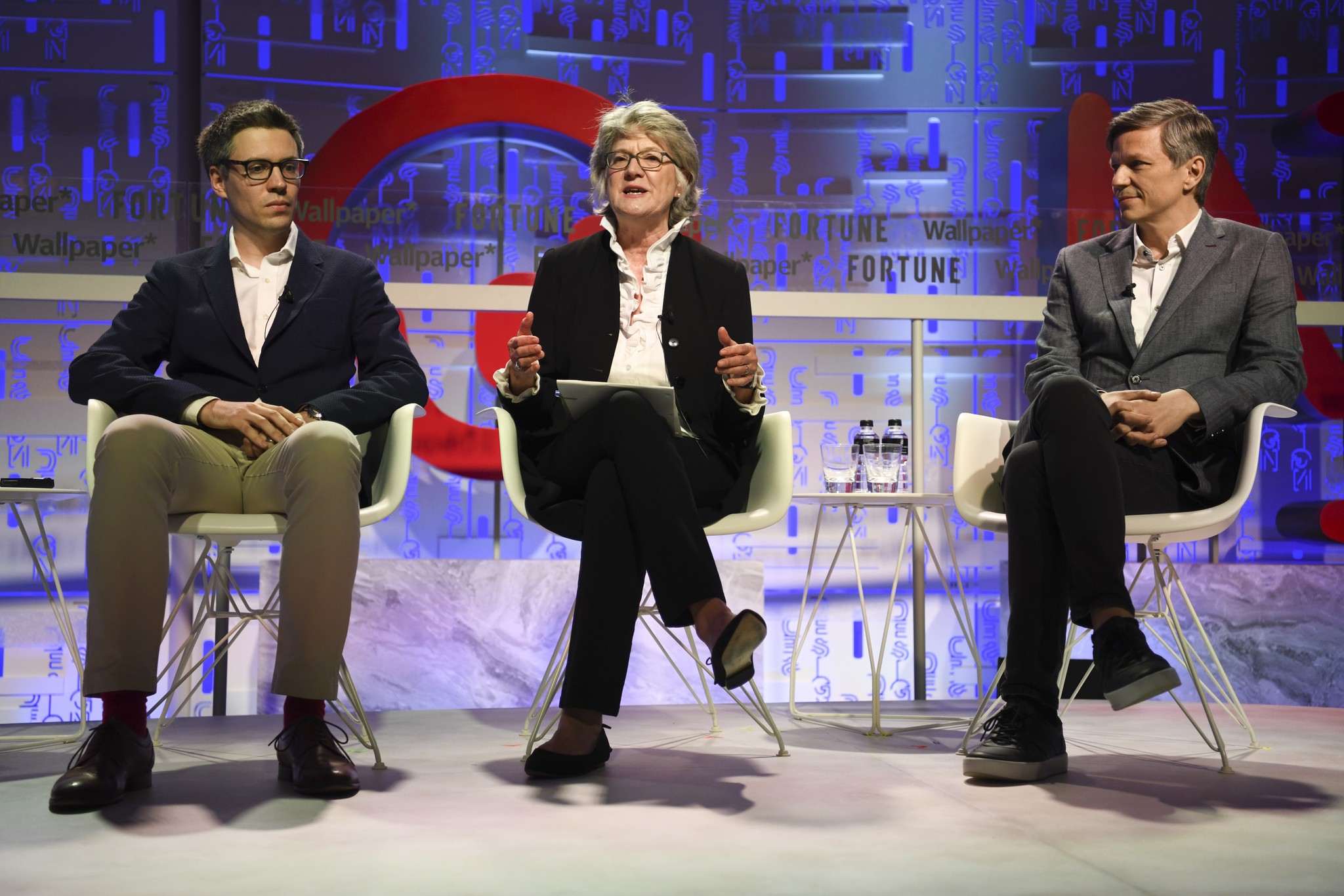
[863,443,902,493]
[820,443,860,493]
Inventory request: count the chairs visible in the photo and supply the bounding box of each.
[952,400,1298,775]
[473,406,794,762]
[86,397,426,771]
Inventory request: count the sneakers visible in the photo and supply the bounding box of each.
[962,695,1067,782]
[1091,615,1182,711]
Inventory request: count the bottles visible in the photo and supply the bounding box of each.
[851,419,880,492]
[882,417,909,493]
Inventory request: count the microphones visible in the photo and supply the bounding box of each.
[658,304,677,326]
[277,284,296,304]
[1121,283,1137,298]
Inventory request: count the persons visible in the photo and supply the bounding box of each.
[50,101,428,814]
[963,99,1304,781]
[493,98,768,776]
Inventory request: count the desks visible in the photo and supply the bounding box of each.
[0,486,89,753]
[788,493,984,738]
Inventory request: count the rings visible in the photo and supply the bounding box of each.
[515,357,527,369]
[266,437,276,444]
[741,365,750,375]
[1140,427,1150,433]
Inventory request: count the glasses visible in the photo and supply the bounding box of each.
[218,158,309,180]
[604,150,678,170]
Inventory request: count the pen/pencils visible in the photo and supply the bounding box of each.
[555,390,578,400]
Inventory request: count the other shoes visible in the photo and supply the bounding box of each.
[524,724,612,777]
[707,608,766,691]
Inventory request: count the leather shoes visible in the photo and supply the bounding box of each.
[48,721,155,806]
[267,717,360,792]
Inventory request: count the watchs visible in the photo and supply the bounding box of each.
[304,405,327,421]
[736,371,757,390]
